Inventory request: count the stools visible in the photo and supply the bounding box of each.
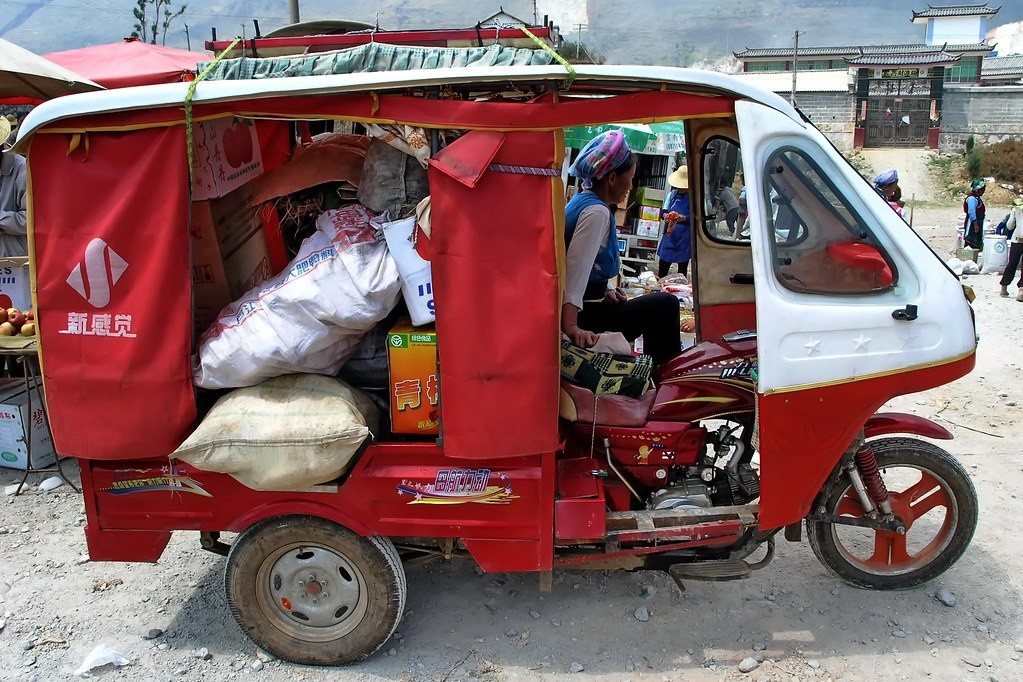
[563,380,656,428]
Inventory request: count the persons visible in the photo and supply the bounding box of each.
[681,317,696,332]
[873,169,907,224]
[0,112,30,257]
[719,172,751,239]
[996,198,1023,302]
[963,178,986,264]
[561,131,681,368]
[657,166,692,278]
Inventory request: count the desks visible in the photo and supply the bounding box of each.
[0,333,81,496]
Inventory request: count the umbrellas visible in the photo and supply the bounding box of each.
[0,36,214,100]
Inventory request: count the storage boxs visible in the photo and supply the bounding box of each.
[194,189,276,337]
[956,248,978,263]
[383,216,435,327]
[617,187,666,235]
[0,257,33,312]
[0,376,71,470]
[386,319,439,434]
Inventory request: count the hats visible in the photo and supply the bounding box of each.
[6,114,18,125]
[0,116,11,146]
[970,179,985,193]
[870,169,898,187]
[567,130,631,190]
[668,165,688,189]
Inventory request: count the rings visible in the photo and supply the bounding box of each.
[686,326,688,328]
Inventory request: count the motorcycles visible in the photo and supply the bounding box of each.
[0,56,983,668]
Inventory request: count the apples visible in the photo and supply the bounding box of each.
[0,307,36,337]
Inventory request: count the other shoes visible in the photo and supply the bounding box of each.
[1017,294,1023,302]
[1001,290,1009,295]
